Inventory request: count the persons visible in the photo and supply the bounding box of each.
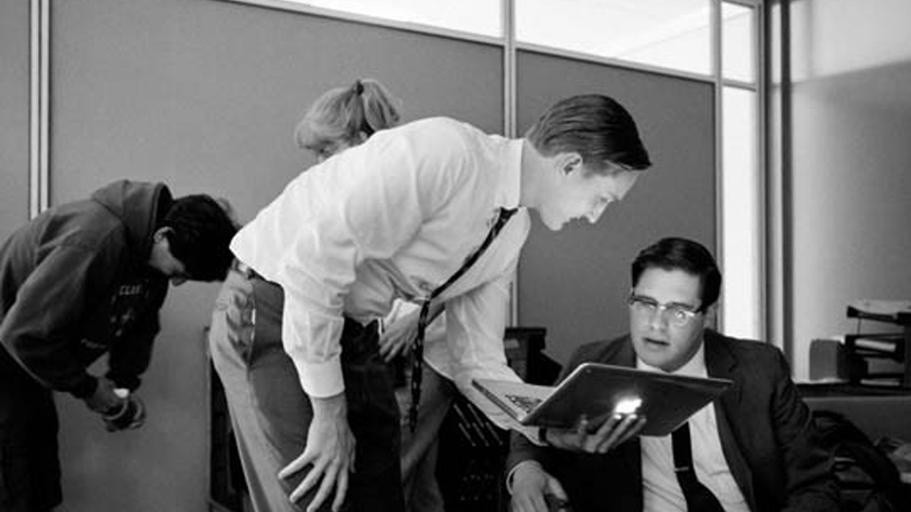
[211,94,648,512]
[506,237,839,512]
[0,182,238,512]
[295,77,451,512]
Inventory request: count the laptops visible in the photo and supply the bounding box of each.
[472,362,733,436]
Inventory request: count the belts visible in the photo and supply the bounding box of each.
[229,257,255,276]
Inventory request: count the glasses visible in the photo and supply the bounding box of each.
[626,291,707,327]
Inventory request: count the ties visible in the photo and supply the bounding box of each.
[671,423,726,512]
[408,207,518,434]
